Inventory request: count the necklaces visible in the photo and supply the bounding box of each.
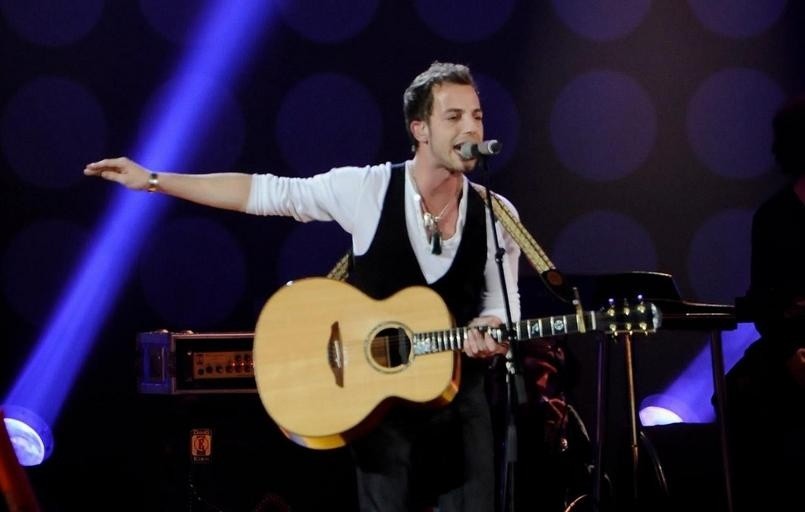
[410,162,464,256]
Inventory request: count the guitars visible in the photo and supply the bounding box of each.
[253,277,662,450]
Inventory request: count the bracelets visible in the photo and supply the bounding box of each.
[147,173,159,194]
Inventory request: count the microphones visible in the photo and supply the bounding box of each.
[460,140,501,159]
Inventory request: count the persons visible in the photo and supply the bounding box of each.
[83,60,522,511]
[749,92,805,382]
